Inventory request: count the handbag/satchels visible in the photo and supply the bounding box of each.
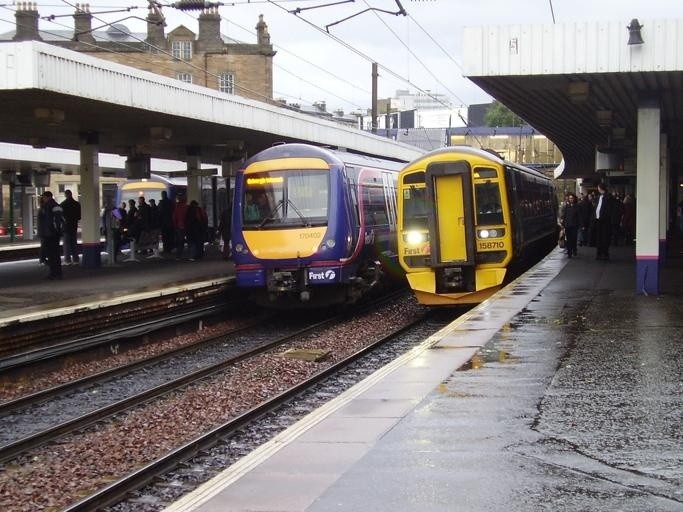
[558,234,568,248]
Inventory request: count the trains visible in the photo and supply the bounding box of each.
[113,172,239,251]
[227,139,415,313]
[394,142,561,311]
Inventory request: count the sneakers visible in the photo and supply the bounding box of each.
[568,252,572,258]
[573,248,577,256]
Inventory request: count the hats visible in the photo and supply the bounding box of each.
[40,192,53,197]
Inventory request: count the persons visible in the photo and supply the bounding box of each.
[519,198,551,218]
[558,182,637,260]
[39,191,66,278]
[480,194,502,213]
[58,188,82,267]
[36,198,49,265]
[100,189,279,264]
